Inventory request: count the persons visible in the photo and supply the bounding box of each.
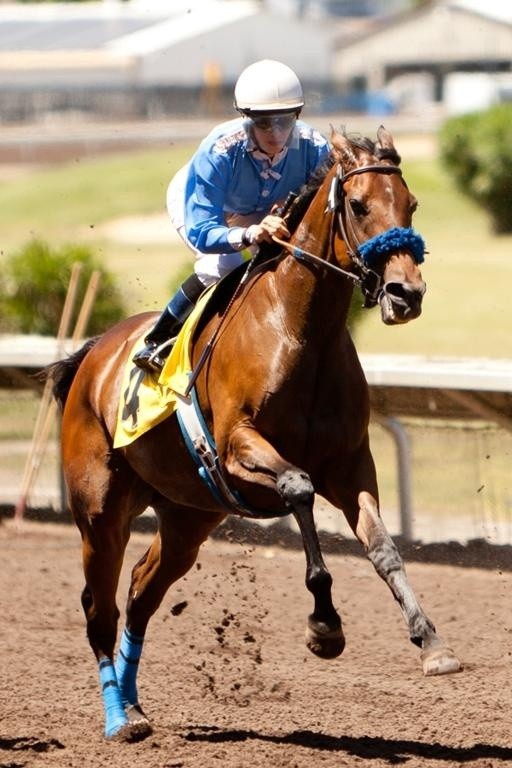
[133,59,341,372]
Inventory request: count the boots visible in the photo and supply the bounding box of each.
[132,273,207,372]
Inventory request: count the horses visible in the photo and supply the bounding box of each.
[28,121,465,747]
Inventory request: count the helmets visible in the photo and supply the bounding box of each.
[233,60,304,119]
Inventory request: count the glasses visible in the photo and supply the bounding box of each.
[244,113,297,131]
